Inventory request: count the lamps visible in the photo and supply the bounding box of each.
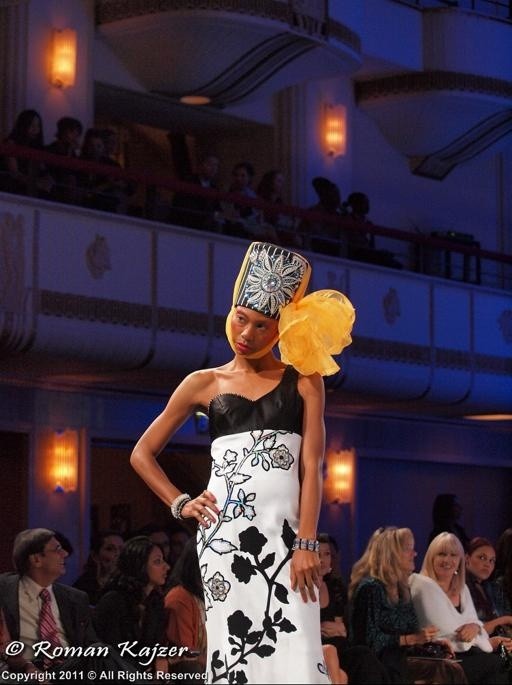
[325,447,355,504]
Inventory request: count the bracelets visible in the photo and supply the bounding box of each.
[171,491,191,526]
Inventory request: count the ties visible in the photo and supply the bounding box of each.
[38,589,65,668]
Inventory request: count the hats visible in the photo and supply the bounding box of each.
[232,242,312,321]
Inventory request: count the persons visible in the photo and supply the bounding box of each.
[337,493,512,685]
[127,237,356,661]
[303,173,383,264]
[0,516,353,685]
[1,107,299,249]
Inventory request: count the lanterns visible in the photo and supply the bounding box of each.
[323,446,356,508]
